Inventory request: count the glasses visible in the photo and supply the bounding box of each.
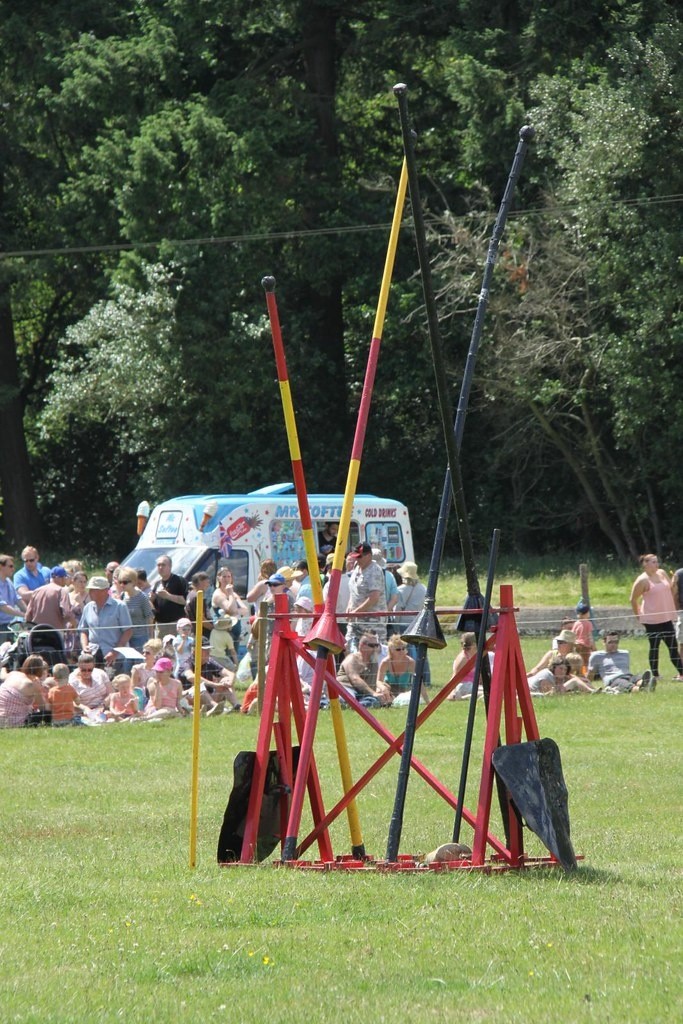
[80,667,93,672]
[23,556,36,562]
[268,583,283,587]
[5,564,13,568]
[181,626,191,631]
[142,651,154,655]
[365,642,379,648]
[392,647,406,651]
[557,641,564,644]
[358,553,368,559]
[119,580,132,585]
[108,569,114,572]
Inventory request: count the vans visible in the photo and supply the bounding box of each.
[109,481,416,637]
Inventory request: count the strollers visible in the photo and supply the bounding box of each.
[4,620,72,679]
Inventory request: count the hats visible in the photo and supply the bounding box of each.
[153,658,172,672]
[214,613,238,630]
[351,542,372,557]
[396,561,419,579]
[576,603,591,615]
[85,577,110,590]
[555,629,576,643]
[276,565,302,580]
[293,596,312,611]
[192,637,214,650]
[264,574,286,584]
[371,549,386,567]
[326,553,334,565]
[50,566,70,578]
[176,617,191,630]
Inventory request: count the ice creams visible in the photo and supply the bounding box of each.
[136,500,150,534]
[199,500,218,531]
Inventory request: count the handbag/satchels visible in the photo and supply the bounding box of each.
[236,652,252,682]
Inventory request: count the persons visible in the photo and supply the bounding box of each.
[338,633,387,709]
[630,554,683,680]
[376,635,431,704]
[527,606,657,696]
[447,634,494,700]
[671,568,683,680]
[319,523,339,554]
[240,559,332,714]
[0,548,248,729]
[318,542,432,690]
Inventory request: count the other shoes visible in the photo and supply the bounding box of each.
[199,701,243,717]
[587,670,683,694]
